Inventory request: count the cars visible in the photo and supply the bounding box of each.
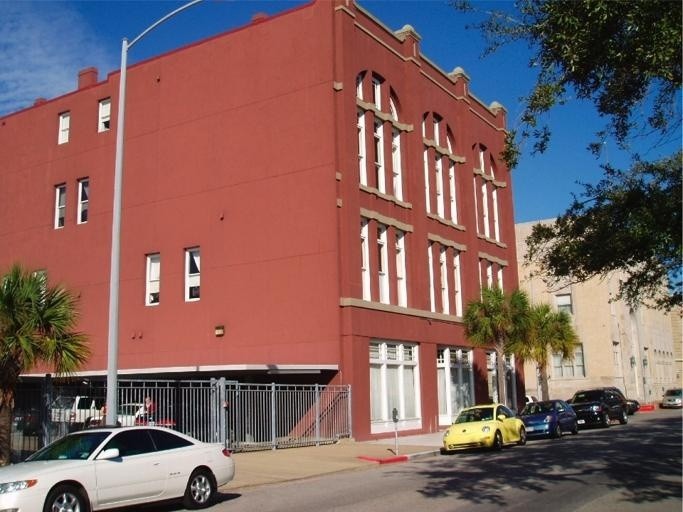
[662,388,683,408]
[0,426,235,512]
[443,387,640,454]
[10,395,176,436]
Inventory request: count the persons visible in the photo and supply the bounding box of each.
[144,397,156,421]
[99,402,106,427]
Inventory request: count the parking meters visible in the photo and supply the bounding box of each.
[392,407,399,456]
